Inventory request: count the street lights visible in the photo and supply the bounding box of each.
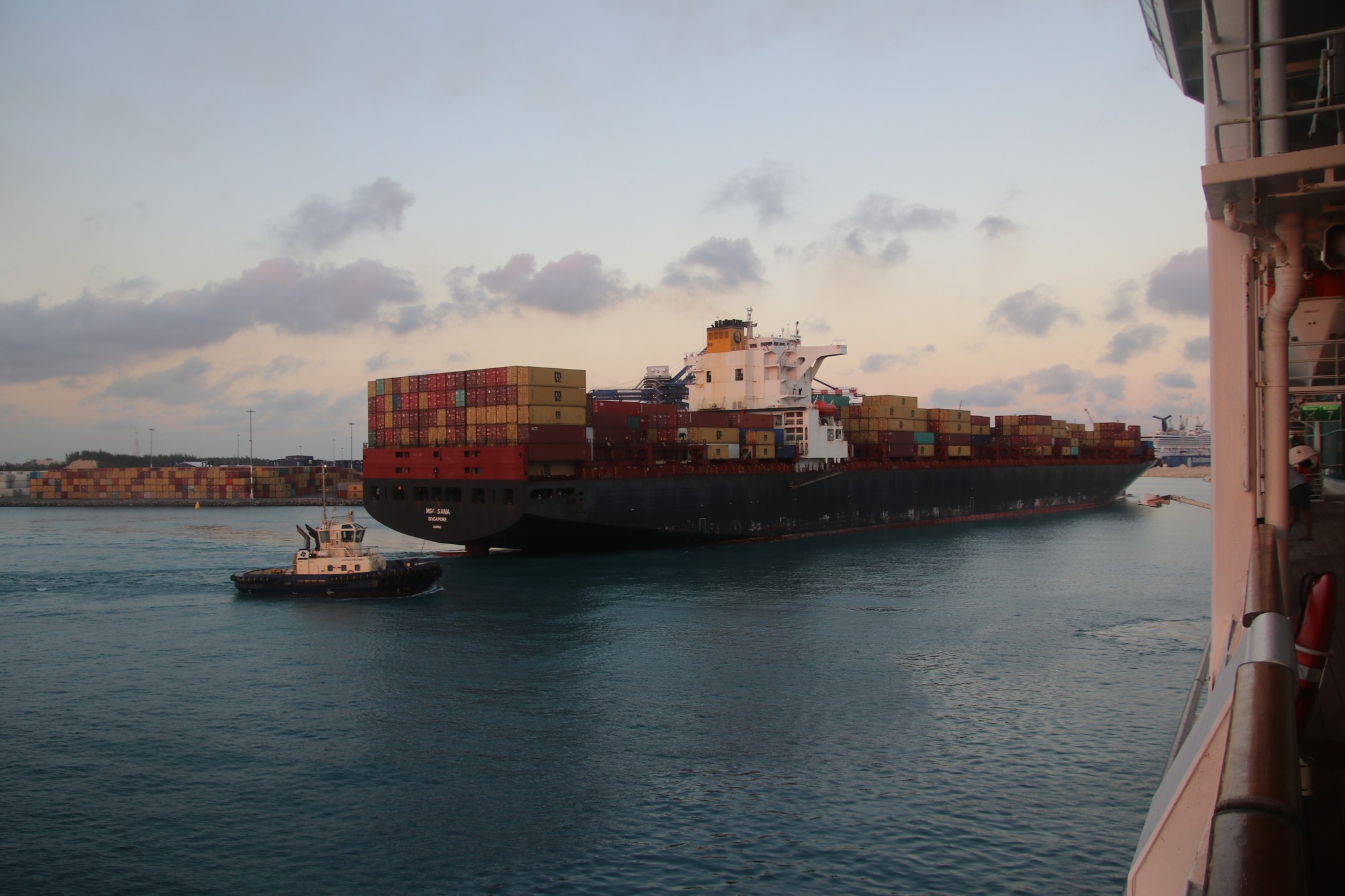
[348,422,355,469]
[148,428,155,468]
[333,439,335,466]
[246,409,256,498]
[237,434,240,466]
[341,448,343,469]
[299,446,302,456]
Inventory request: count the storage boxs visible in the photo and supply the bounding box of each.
[0,466,363,499]
[367,366,1155,458]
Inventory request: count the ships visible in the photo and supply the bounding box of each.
[362,305,1159,552]
[1140,414,1210,468]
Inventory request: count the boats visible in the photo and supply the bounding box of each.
[814,400,836,416]
[230,461,444,601]
